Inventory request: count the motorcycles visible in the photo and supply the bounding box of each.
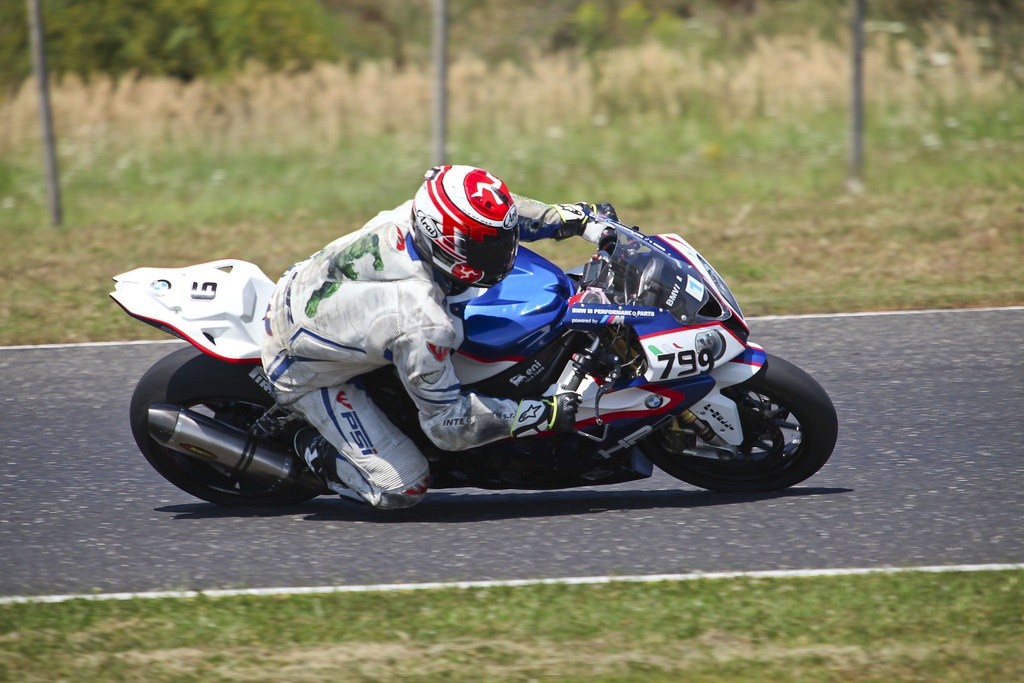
[108,216,838,510]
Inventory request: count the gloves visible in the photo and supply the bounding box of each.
[510,391,581,439]
[549,201,619,242]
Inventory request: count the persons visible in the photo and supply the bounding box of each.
[260,163,619,510]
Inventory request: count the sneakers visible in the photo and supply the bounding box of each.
[295,427,350,491]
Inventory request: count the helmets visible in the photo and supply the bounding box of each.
[409,164,518,287]
